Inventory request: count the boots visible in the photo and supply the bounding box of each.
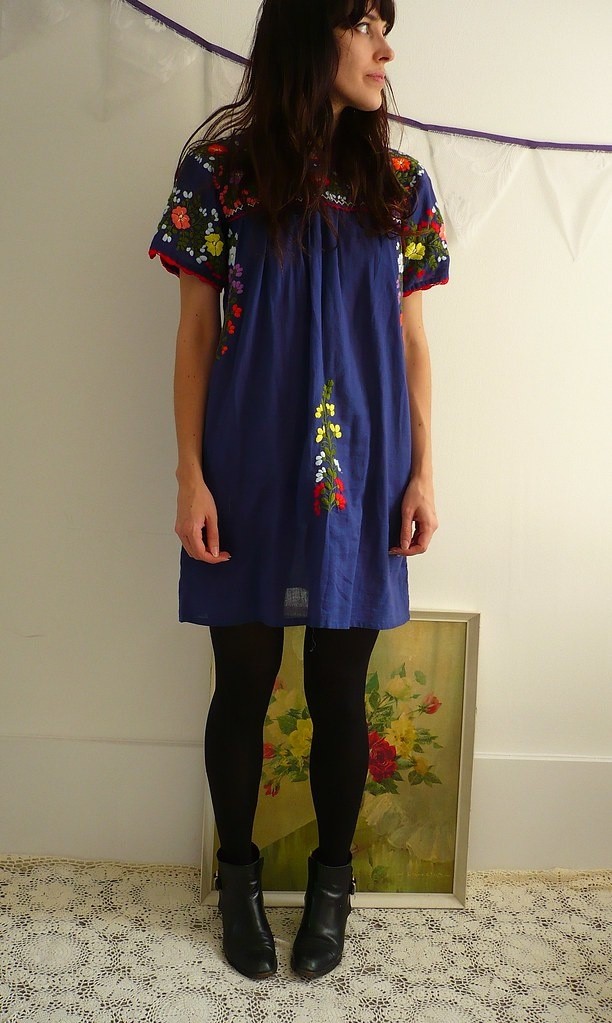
[291,847,357,978]
[211,842,278,979]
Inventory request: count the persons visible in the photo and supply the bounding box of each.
[148,1,451,980]
